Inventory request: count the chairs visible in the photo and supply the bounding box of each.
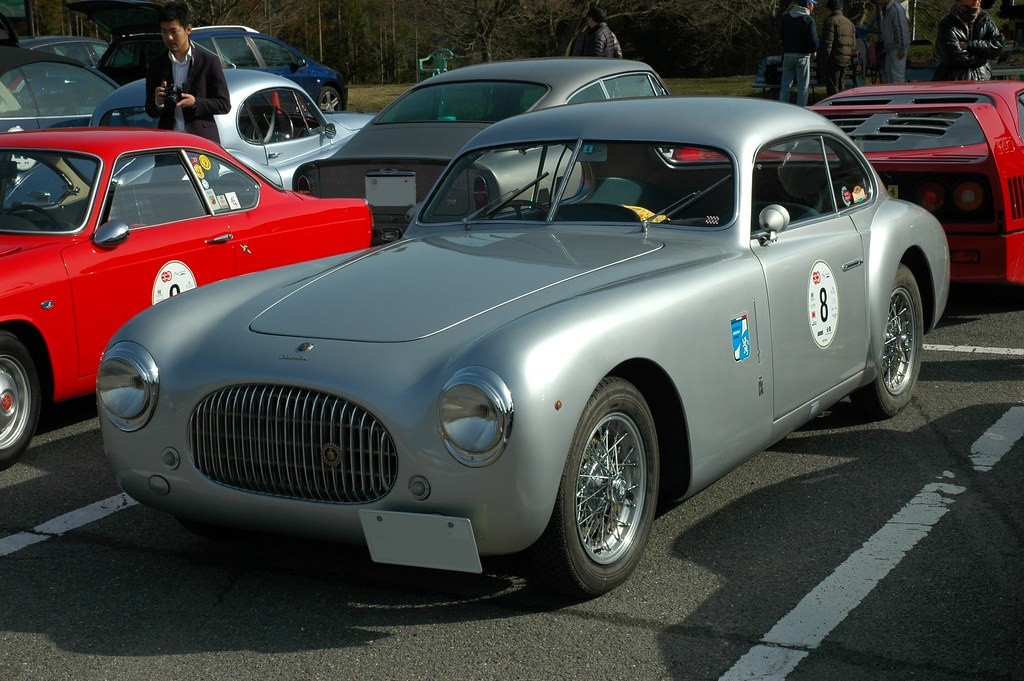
[583,175,645,212]
[442,83,484,120]
[490,82,528,118]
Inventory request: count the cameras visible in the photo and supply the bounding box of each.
[163,84,183,106]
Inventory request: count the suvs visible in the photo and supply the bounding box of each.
[95,24,349,116]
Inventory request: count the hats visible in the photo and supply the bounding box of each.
[800,0,817,4]
[826,0,843,9]
[588,6,607,21]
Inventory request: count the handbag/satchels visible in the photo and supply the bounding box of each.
[610,30,623,60]
[764,52,798,85]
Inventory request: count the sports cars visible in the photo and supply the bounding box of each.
[666,78,1024,285]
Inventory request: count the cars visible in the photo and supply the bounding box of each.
[82,67,380,206]
[96,95,953,600]
[0,39,122,130]
[289,57,672,254]
[1,126,375,470]
[7,35,134,115]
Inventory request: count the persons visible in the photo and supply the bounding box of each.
[572,4,623,60]
[821,0,858,97]
[931,0,1005,80]
[146,3,230,185]
[778,0,818,107]
[873,0,910,84]
